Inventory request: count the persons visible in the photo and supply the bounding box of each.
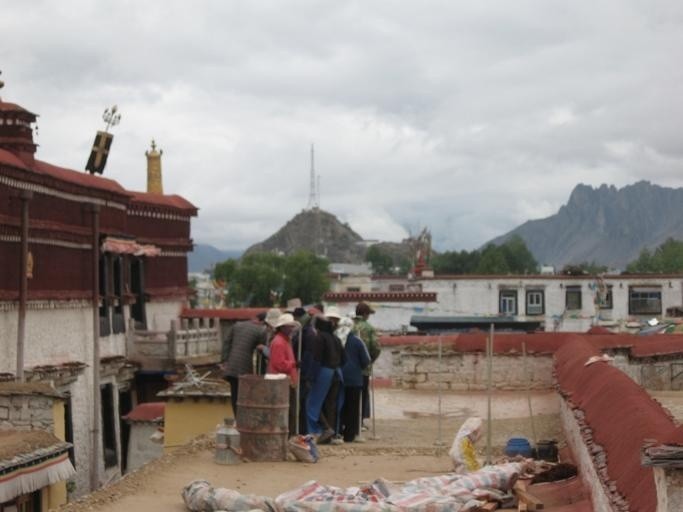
[220,298,381,444]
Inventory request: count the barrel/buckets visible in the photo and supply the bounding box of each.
[504,438,532,458]
[537,437,559,460]
[235,375,290,463]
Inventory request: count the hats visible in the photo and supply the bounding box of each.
[265,298,302,332]
[356,303,375,314]
[325,306,342,319]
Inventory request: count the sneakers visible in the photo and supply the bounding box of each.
[317,428,335,444]
